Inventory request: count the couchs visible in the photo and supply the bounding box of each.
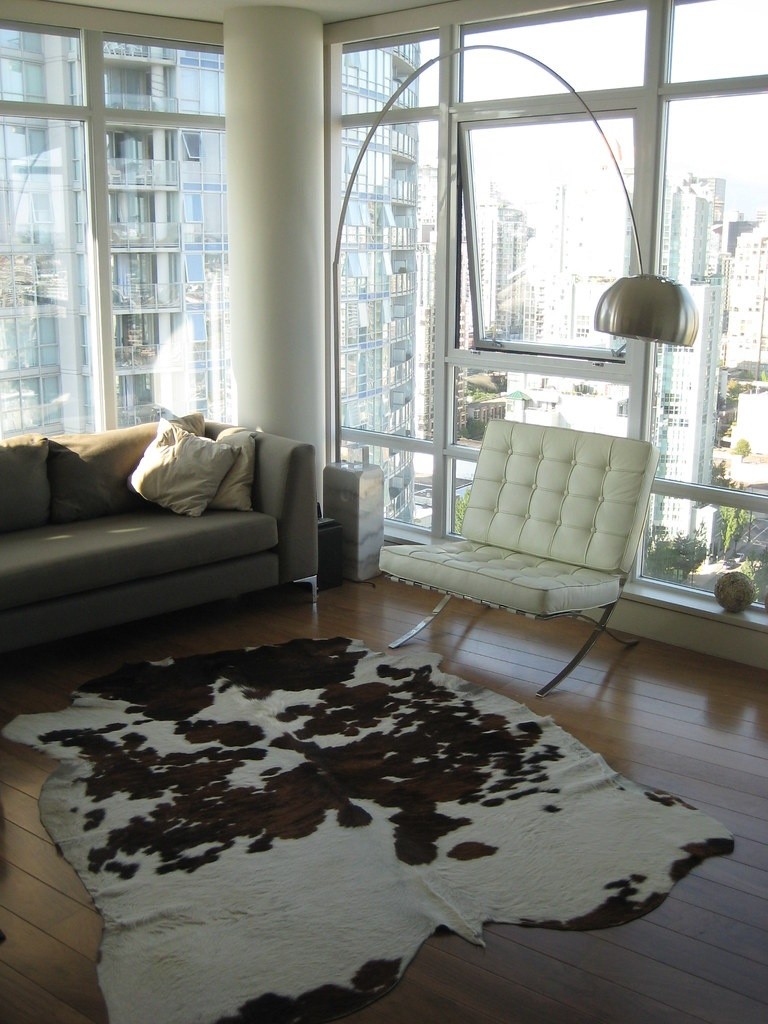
[0,421,320,654]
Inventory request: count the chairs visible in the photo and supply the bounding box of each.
[379,417,660,693]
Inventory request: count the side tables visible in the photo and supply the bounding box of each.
[307,521,343,589]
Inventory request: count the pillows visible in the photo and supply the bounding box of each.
[208,426,258,512]
[126,417,242,518]
[52,412,205,525]
[0,432,53,532]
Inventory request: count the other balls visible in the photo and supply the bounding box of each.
[713,571,756,612]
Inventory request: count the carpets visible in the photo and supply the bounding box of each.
[0,635,735,1024]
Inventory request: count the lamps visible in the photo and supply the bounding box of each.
[319,43,699,583]
